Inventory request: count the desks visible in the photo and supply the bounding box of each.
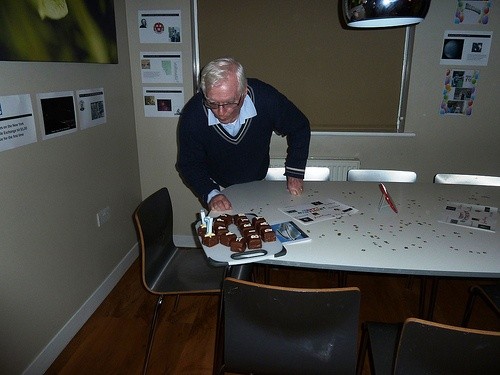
[208,180,500,323]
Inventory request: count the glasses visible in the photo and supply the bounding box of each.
[203,92,242,109]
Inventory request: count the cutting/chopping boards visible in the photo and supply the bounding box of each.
[190,212,287,267]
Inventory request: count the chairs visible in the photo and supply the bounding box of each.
[343,170,426,314]
[368,284,500,375]
[429,174,500,322]
[264,166,343,289]
[133,187,228,375]
[217,277,361,375]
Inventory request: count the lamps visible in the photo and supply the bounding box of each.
[342,0,431,28]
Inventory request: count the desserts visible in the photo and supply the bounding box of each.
[198,213,276,253]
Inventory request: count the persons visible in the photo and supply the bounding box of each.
[140,19,147,28]
[80,101,85,111]
[174,57,311,212]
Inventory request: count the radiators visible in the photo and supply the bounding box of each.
[269,159,361,181]
